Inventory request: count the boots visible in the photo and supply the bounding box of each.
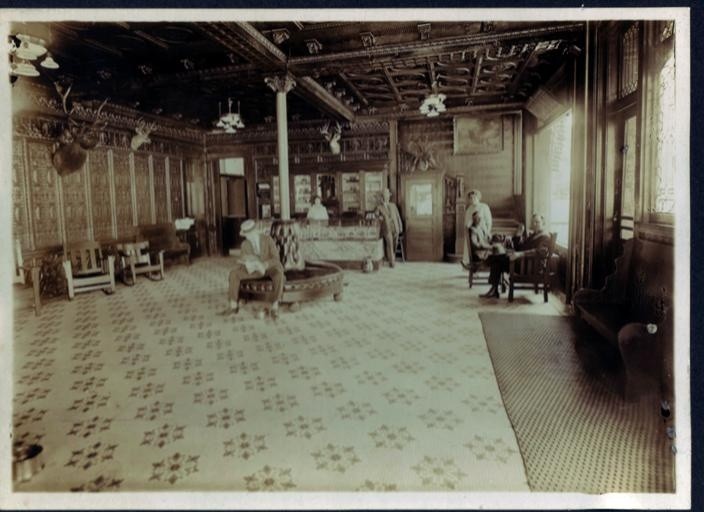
[479,288,500,300]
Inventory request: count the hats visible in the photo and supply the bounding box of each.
[380,188,393,197]
[239,219,260,236]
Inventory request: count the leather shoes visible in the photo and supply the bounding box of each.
[264,308,279,322]
[218,306,238,319]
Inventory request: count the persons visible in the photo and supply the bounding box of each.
[298,179,309,203]
[223,220,284,320]
[461,214,551,299]
[306,195,329,221]
[375,188,402,267]
[463,189,493,269]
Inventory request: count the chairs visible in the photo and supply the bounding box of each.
[458,225,558,303]
[59,222,193,300]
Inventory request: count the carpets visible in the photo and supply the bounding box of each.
[478,310,676,493]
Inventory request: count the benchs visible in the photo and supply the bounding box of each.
[238,262,345,313]
[572,268,666,403]
